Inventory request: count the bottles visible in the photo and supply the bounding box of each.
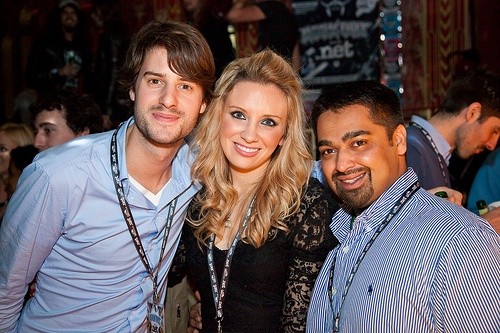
[476,200,488,215]
[434,191,448,198]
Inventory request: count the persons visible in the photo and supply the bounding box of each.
[165,48,338,333]
[0,21,463,333]
[186,80,500,333]
[403,66,500,237]
[0,0,464,333]
